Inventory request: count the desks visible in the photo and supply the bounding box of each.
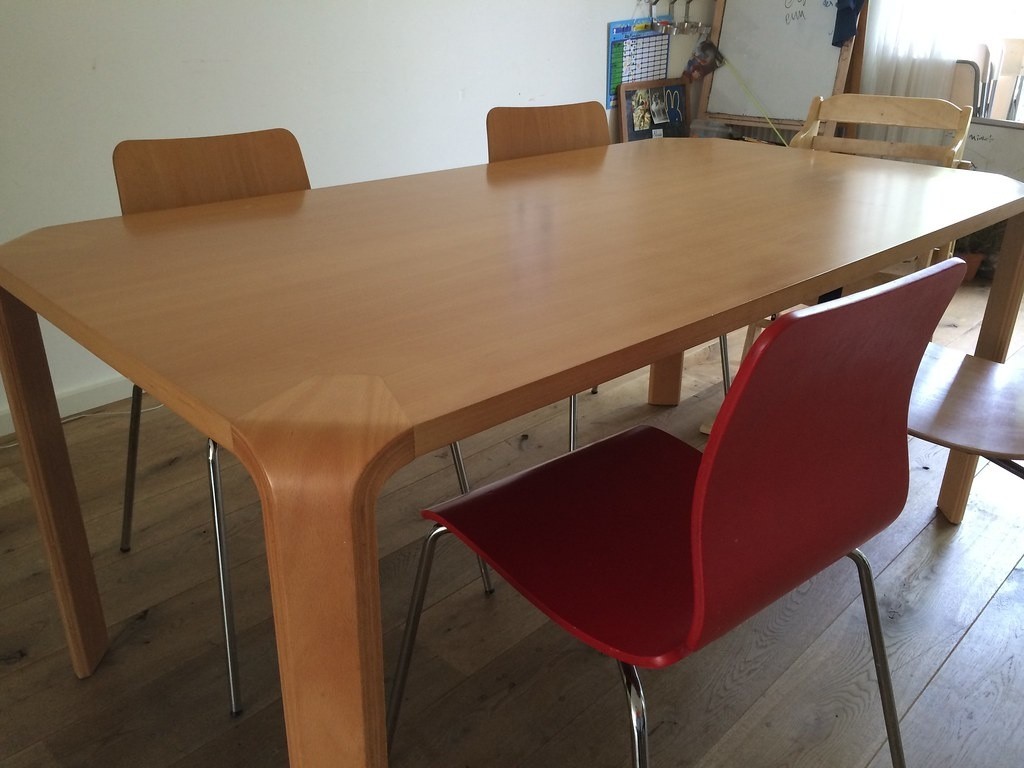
[1,135,1024,768]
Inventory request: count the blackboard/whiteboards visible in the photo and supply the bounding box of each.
[696,0,862,153]
[616,76,692,145]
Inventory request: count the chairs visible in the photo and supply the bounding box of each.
[113,127,503,729]
[377,250,1024,768]
[484,98,740,461]
[702,90,975,443]
